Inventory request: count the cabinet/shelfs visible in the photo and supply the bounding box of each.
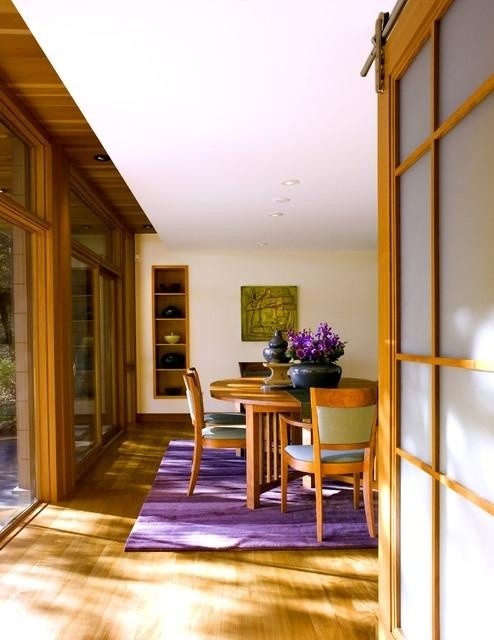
[151,265,190,400]
[71,266,95,398]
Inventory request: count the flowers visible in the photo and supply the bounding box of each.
[286,321,344,362]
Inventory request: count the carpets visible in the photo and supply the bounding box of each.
[123,440,378,552]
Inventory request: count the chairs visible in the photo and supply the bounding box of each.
[182,362,379,543]
